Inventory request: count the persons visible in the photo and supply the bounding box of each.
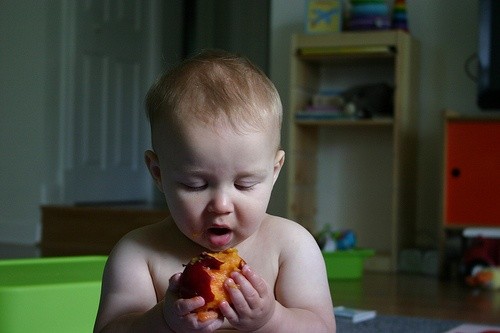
[93,53,337,333]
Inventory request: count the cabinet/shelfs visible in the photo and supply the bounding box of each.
[282,28,419,272]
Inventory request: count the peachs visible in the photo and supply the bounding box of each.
[176,249,246,321]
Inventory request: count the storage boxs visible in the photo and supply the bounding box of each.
[323,247,373,281]
[0,257,108,333]
